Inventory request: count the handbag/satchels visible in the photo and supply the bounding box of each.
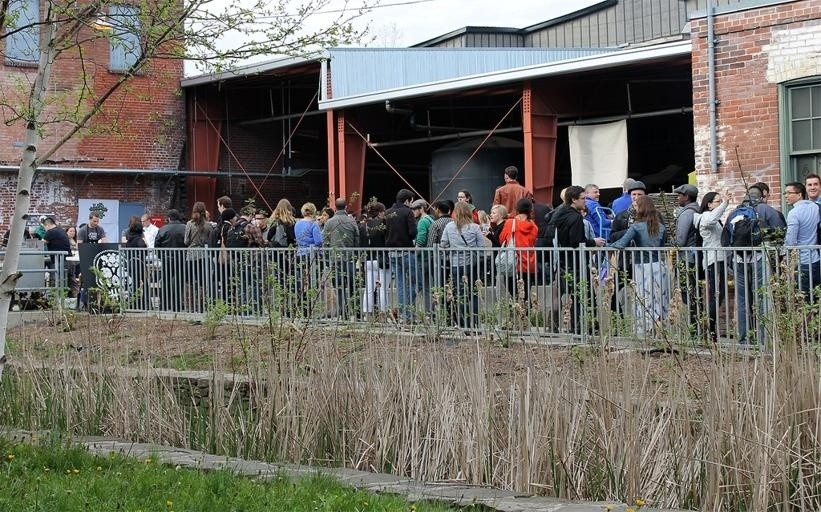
[271,225,288,247]
[218,244,230,265]
[376,256,389,268]
[475,256,491,272]
[496,245,518,277]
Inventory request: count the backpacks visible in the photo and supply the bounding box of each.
[728,202,762,257]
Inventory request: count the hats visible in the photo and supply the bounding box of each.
[222,208,236,222]
[628,182,647,193]
[411,199,427,209]
[674,184,698,197]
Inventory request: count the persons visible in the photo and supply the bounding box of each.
[545,175,820,336]
[2,212,107,312]
[120,190,552,336]
[493,166,533,214]
